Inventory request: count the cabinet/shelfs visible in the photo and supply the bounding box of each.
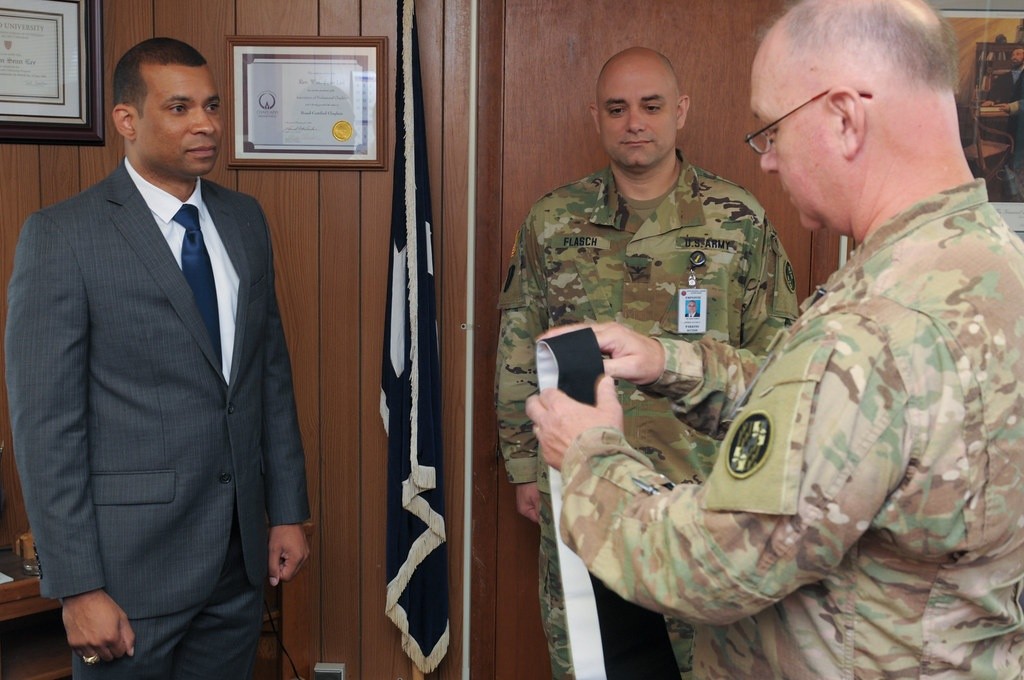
[0,547,73,680]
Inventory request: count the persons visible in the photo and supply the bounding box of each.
[4,37,312,680]
[985,49,1024,115]
[527,1,1024,680]
[685,299,700,317]
[496,42,802,680]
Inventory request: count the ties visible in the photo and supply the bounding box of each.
[171,204,222,376]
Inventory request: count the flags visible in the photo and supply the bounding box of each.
[378,0,452,674]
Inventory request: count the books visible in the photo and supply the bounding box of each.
[975,45,1009,89]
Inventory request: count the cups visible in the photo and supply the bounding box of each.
[16,534,39,576]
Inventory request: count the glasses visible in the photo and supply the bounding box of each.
[745,86,873,155]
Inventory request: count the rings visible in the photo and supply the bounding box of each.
[80,654,99,666]
